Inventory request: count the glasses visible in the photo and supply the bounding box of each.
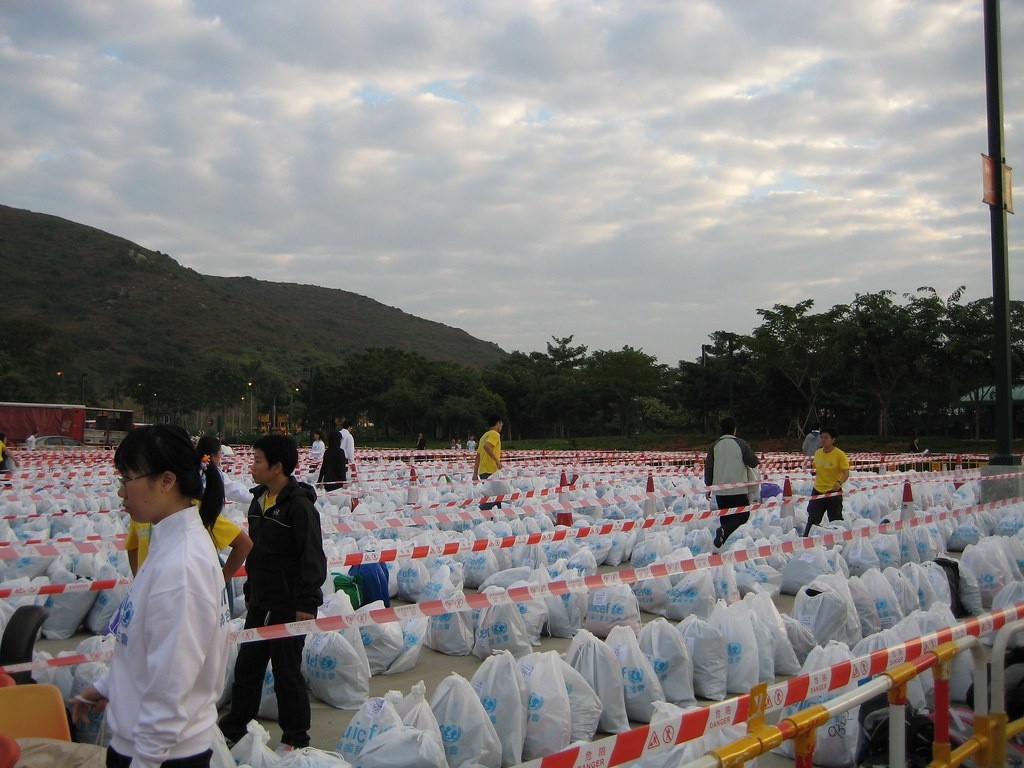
[117,467,168,488]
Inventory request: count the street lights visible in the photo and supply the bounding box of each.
[241,397,247,434]
[81,372,90,405]
[248,382,252,433]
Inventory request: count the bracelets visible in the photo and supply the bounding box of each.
[837,481,841,486]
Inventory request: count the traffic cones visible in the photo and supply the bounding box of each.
[953,453,966,490]
[780,475,796,519]
[406,468,419,506]
[899,478,916,522]
[556,470,574,527]
[643,472,657,518]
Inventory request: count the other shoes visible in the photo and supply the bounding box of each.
[714,527,725,547]
[275,743,301,757]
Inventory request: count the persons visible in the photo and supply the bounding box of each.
[466,435,477,450]
[416,432,426,450]
[197,435,254,504]
[219,433,327,754]
[0,433,19,474]
[804,428,850,537]
[802,423,823,455]
[69,423,230,768]
[26,430,38,451]
[448,439,461,449]
[124,498,253,583]
[316,431,348,492]
[309,430,325,473]
[705,417,758,548]
[910,437,920,453]
[472,414,503,509]
[339,420,355,464]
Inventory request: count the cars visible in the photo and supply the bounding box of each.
[33,436,91,451]
